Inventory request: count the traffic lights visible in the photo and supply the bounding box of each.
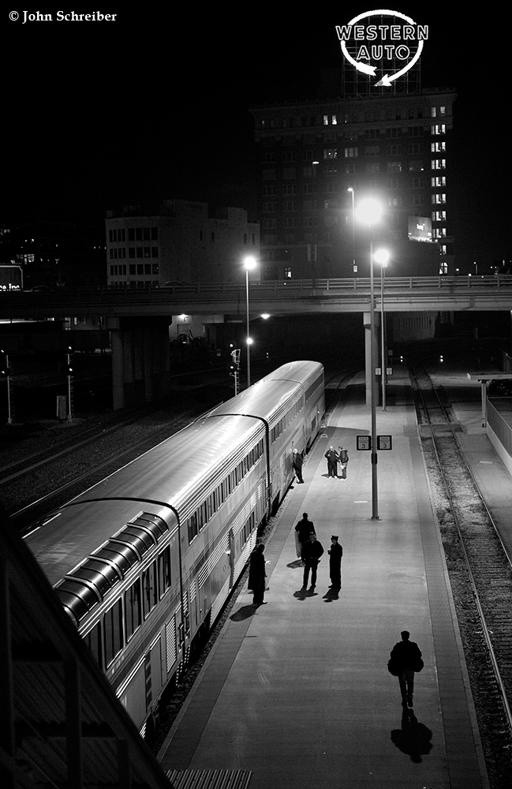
[65,363,77,376]
[226,361,237,373]
[65,343,75,354]
[0,368,10,378]
[0,345,8,356]
[227,341,237,351]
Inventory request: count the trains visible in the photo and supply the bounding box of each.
[21,359,328,736]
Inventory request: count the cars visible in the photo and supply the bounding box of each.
[155,279,198,292]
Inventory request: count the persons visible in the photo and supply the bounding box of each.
[301,530,323,589]
[388,630,424,708]
[324,443,350,479]
[295,513,317,558]
[292,448,304,484]
[327,534,344,590]
[248,542,268,604]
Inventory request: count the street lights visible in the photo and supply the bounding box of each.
[240,253,271,388]
[358,193,390,523]
[371,245,393,412]
[345,185,358,261]
